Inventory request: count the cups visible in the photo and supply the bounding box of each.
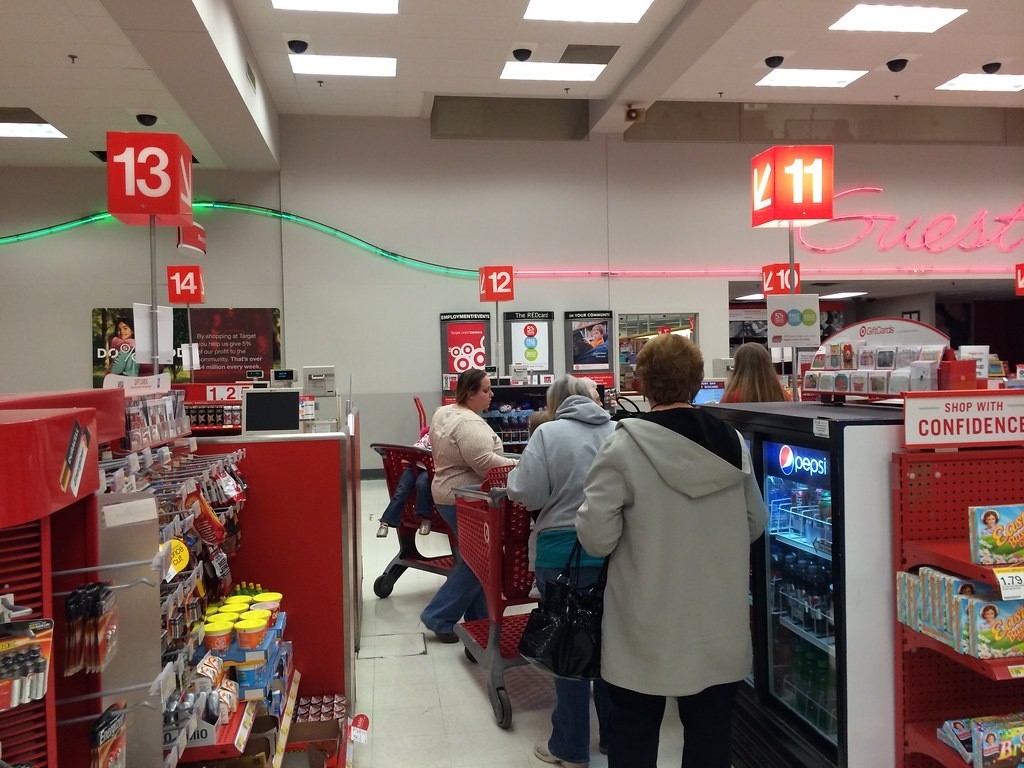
[203,592,282,652]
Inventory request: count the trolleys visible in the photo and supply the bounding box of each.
[451,465,545,729]
[369,440,457,598]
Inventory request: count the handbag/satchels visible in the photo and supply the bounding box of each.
[516,540,610,682]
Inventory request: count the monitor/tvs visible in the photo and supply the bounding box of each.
[242,389,301,436]
[690,377,729,404]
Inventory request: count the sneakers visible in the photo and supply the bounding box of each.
[599,745,608,754]
[419,519,431,535]
[376,523,388,538]
[535,739,589,768]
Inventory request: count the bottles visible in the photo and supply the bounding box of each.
[779,555,837,734]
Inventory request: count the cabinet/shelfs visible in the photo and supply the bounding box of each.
[892,452,1024,768]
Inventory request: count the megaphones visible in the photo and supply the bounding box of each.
[624,107,645,123]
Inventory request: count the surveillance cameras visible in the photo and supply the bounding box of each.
[887,59,908,72]
[513,49,531,62]
[765,56,784,68]
[287,40,308,54]
[982,63,1001,74]
[136,114,157,126]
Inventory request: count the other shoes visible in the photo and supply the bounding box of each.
[420,615,459,643]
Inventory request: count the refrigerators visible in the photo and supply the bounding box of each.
[691,402,903,768]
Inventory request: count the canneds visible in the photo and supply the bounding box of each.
[184,405,242,426]
[771,487,831,545]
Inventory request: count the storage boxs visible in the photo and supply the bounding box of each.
[162,610,341,767]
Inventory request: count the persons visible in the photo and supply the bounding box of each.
[420,369,519,642]
[506,334,771,768]
[105,318,139,377]
[581,324,605,350]
[718,342,792,403]
[377,425,436,538]
